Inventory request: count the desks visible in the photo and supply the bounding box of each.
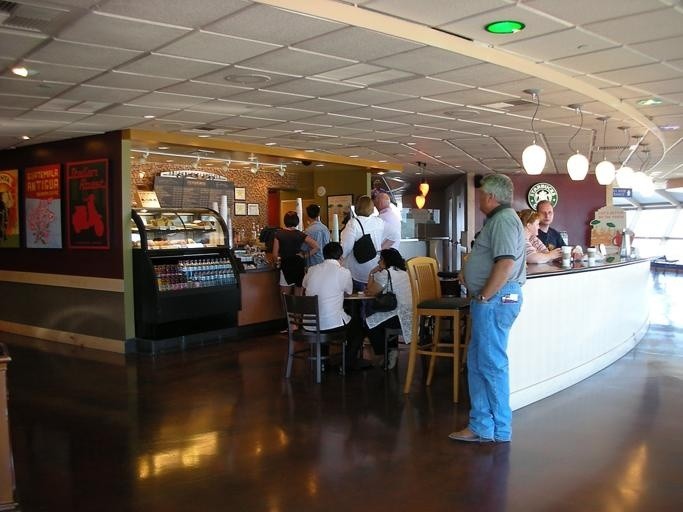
[325,290,376,371]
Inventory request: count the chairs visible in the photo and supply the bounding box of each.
[283,294,347,382]
[404,256,471,404]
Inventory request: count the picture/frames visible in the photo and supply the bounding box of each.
[326,194,353,233]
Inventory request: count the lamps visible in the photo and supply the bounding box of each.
[128,148,287,178]
[415,161,429,210]
[521,88,654,197]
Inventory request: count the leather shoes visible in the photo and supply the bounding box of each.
[449,427,491,442]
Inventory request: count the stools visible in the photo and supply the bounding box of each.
[383,326,403,372]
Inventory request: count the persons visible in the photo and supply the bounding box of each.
[370,179,386,200]
[535,199,585,263]
[270,192,420,374]
[448,173,527,443]
[516,209,563,264]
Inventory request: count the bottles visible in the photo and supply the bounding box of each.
[620,232,627,263]
[232,221,263,243]
[187,270,236,288]
[152,265,187,293]
[178,257,233,279]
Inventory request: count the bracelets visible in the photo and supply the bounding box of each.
[476,294,486,301]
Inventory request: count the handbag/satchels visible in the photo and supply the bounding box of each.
[374,293,397,311]
[353,234,376,263]
[282,258,301,285]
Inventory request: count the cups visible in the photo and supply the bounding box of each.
[587,248,596,267]
[295,198,302,233]
[332,214,339,242]
[211,201,218,212]
[227,218,232,250]
[219,195,227,226]
[560,246,573,269]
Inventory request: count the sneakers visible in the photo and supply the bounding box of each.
[388,350,398,369]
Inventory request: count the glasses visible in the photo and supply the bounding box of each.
[471,231,480,248]
[528,211,536,219]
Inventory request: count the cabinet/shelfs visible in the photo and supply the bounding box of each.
[130,209,242,339]
